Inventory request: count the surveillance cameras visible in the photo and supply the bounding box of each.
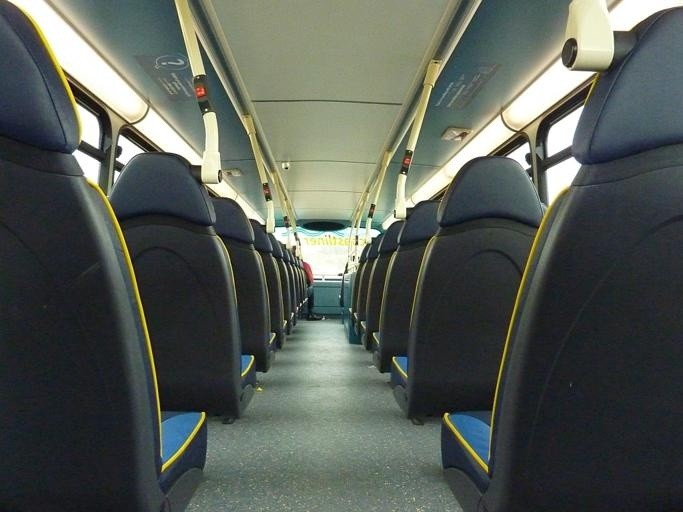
[282,163,289,170]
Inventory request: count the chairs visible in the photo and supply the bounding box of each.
[344,1,682,509]
[1,1,320,510]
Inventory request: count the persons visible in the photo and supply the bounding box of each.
[299,260,321,320]
